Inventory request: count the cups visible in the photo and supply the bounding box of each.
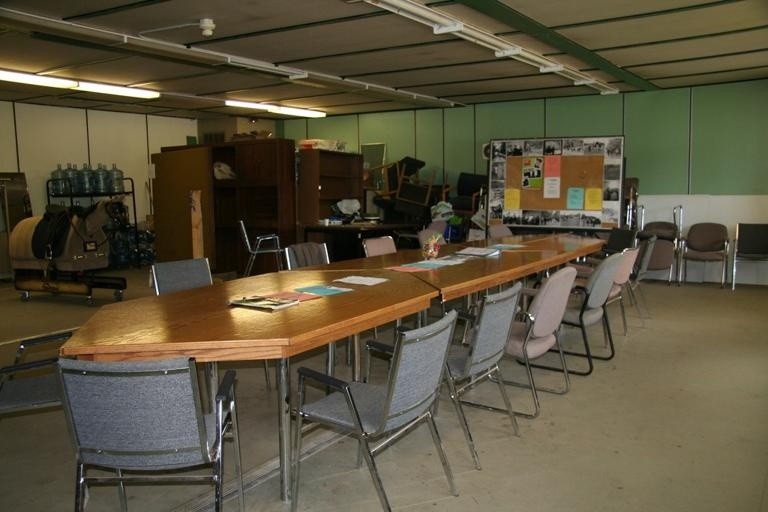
[324,219,329,226]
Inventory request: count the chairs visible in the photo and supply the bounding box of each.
[636,223,679,287]
[366,282,524,471]
[150,259,272,405]
[488,223,539,289]
[633,205,682,267]
[53,357,246,512]
[284,243,352,385]
[373,156,426,195]
[397,164,434,223]
[292,309,459,511]
[420,230,479,309]
[680,222,729,286]
[467,267,578,420]
[426,183,451,203]
[532,254,623,376]
[445,172,488,226]
[568,262,595,277]
[362,236,419,339]
[604,228,636,254]
[539,247,640,346]
[0,333,71,415]
[732,223,768,289]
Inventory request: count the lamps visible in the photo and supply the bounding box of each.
[0,68,161,100]
[226,99,327,119]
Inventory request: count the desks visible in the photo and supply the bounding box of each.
[303,218,395,257]
[57,233,606,502]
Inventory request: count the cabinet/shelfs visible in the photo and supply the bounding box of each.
[46,177,137,229]
[0,173,26,282]
[299,147,365,246]
[152,138,298,273]
